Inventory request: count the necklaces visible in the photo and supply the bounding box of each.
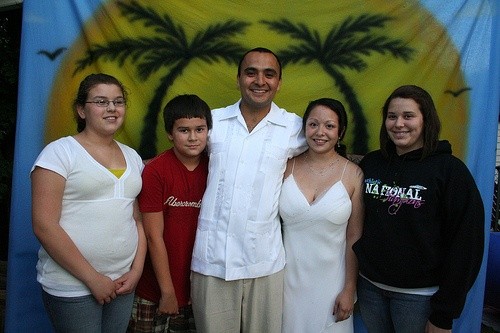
[303,153,339,174]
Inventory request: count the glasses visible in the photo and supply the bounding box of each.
[84,98,125,107]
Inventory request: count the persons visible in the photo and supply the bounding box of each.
[30,73,147,332]
[358,85,486,333]
[143,48,364,333]
[126,94,213,333]
[278,97,366,333]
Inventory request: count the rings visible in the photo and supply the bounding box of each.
[349,314,352,317]
